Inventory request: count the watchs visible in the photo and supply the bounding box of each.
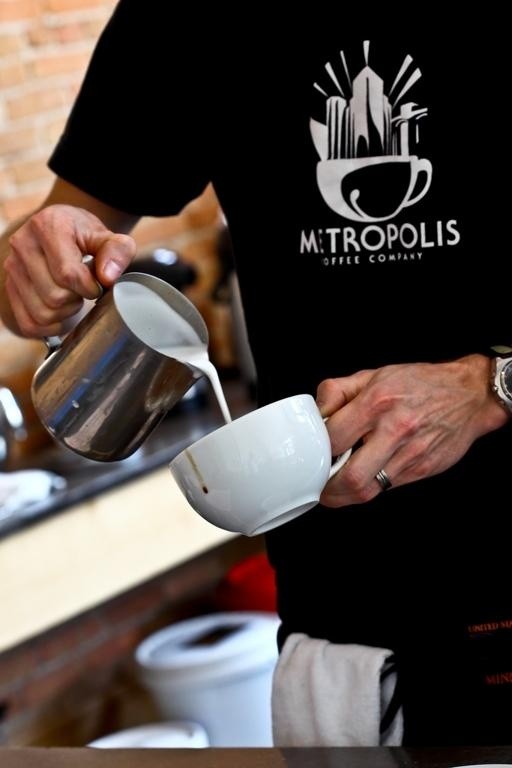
[482,343,512,415]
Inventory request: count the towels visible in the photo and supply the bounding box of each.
[272,632,405,749]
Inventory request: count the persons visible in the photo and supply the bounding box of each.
[1,2,511,752]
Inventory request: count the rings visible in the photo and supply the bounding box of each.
[374,466,394,490]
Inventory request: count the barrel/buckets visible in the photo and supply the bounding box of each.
[84,720,209,750]
[127,612,283,748]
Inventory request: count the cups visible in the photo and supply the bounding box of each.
[168,394,358,539]
[30,254,213,461]
